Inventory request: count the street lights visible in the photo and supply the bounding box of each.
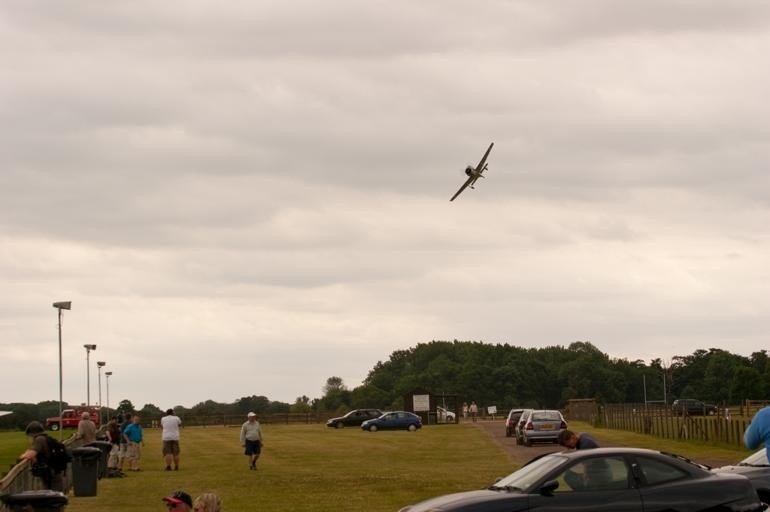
[52,299,72,443]
[84,342,113,429]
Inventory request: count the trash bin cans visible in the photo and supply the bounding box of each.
[429,412,436,424]
[0,489,69,512]
[69,434,112,497]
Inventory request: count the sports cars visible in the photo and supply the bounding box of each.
[394,446,760,511]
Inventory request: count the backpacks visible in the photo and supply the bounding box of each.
[44,433,70,477]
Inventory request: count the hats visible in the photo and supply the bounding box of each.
[247,412,257,418]
[162,491,193,508]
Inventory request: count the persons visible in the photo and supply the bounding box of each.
[193,493,222,512]
[74,411,145,471]
[744,405,770,465]
[558,430,612,485]
[162,490,193,512]
[161,409,182,471]
[18,421,68,494]
[240,412,265,470]
[469,400,478,422]
[462,401,469,420]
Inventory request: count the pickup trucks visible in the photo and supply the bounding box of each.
[45,406,102,432]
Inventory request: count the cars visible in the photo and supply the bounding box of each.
[325,408,383,431]
[524,410,569,443]
[436,406,455,423]
[711,447,769,502]
[361,409,423,434]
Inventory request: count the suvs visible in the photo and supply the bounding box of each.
[671,398,718,418]
[504,408,535,435]
[515,411,532,443]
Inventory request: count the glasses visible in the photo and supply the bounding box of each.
[166,502,176,508]
